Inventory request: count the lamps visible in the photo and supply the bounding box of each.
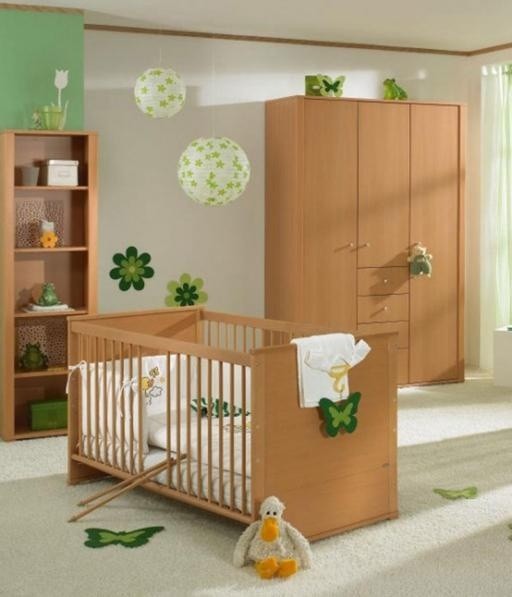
[177,34,253,209]
[133,32,187,120]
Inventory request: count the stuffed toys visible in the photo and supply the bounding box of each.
[233,496,312,579]
[406,242,432,278]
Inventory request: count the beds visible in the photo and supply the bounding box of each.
[64,304,402,535]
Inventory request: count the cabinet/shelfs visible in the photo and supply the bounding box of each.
[1,128,99,443]
[264,94,469,388]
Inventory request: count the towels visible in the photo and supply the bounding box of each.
[291,333,356,410]
